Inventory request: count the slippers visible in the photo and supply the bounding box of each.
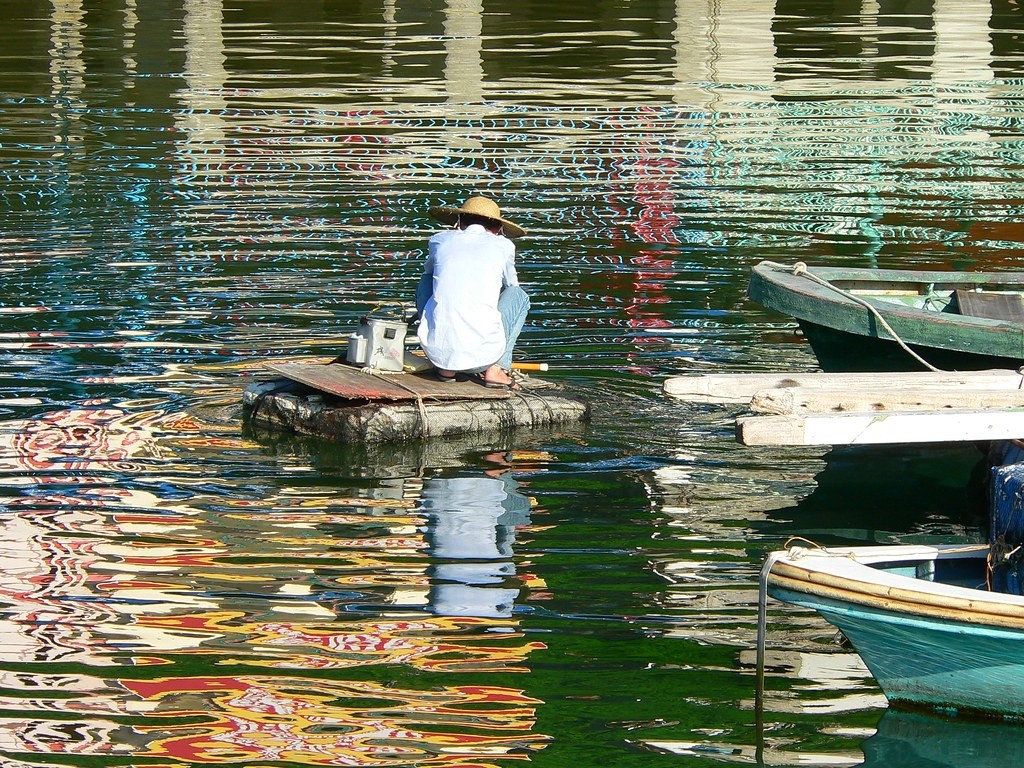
[481,376,522,391]
[437,371,457,383]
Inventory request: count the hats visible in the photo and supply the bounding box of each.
[429,197,527,238]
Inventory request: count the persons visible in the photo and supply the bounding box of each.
[416,197,531,390]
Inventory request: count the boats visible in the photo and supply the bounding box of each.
[761,524,1024,726]
[745,261,1024,373]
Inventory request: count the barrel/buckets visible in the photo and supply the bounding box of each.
[347,300,408,374]
